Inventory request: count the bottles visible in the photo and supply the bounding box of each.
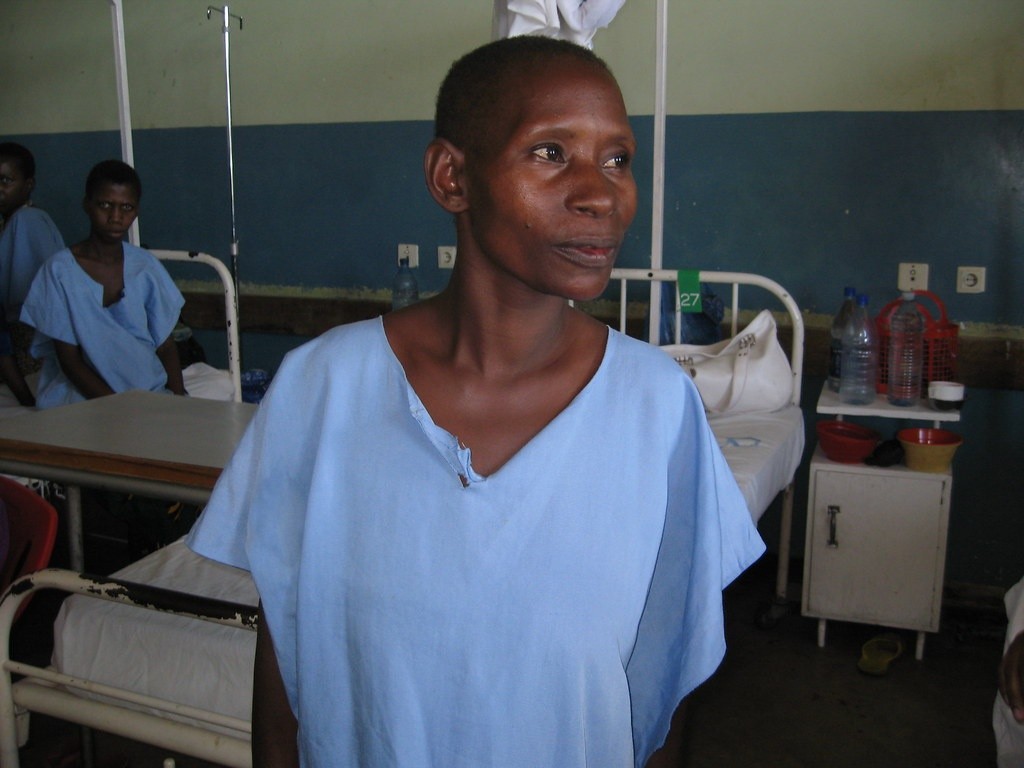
[828,287,857,393]
[840,296,879,405]
[889,292,922,405]
[392,257,419,311]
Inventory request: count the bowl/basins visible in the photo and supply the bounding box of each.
[895,428,964,474]
[815,420,879,464]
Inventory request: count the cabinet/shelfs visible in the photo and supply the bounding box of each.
[801,384,960,663]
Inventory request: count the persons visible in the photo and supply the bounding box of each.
[989,575,1024,768]
[182,35,766,768]
[19,160,190,535]
[0,143,65,372]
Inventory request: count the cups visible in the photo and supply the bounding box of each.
[927,380,965,412]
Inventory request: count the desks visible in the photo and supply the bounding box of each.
[1,389,262,568]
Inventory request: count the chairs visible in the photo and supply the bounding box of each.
[2,479,58,625]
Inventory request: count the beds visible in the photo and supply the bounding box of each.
[0,269,807,768]
[3,248,242,402]
